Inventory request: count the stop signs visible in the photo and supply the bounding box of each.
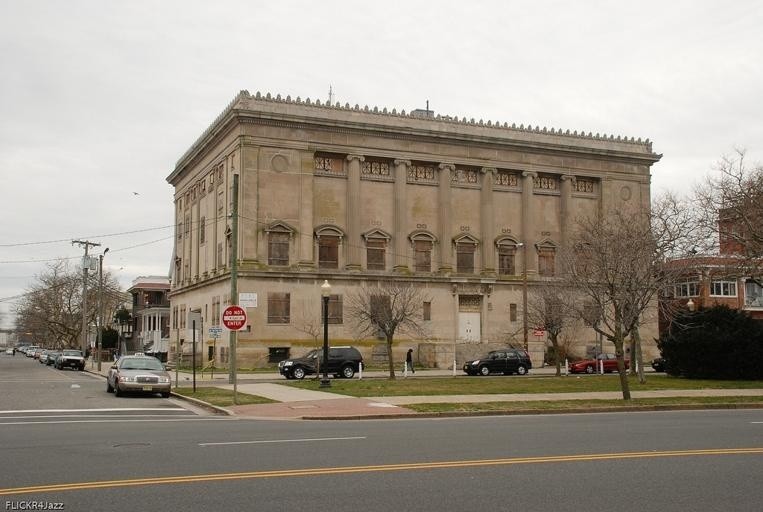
[222,305,247,330]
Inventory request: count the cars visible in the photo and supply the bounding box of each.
[279,346,364,380]
[6,345,85,370]
[106,353,170,397]
[463,348,532,376]
[570,353,630,374]
[651,358,665,372]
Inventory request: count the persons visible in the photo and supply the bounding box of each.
[403,349,415,373]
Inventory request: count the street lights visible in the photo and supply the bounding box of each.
[319,279,332,387]
